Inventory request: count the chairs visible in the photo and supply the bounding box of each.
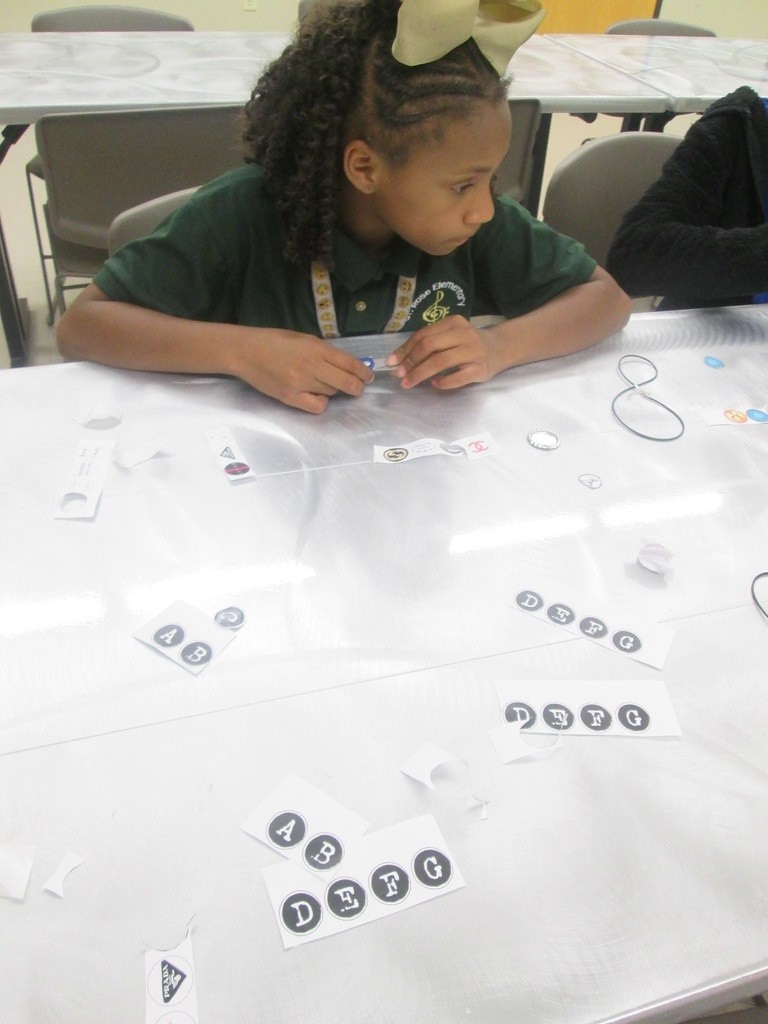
[544,129,683,270]
[25,5,196,325]
[569,22,718,147]
[35,104,245,315]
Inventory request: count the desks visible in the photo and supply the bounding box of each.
[0,30,668,370]
[0,303,768,1024]
[544,33,768,134]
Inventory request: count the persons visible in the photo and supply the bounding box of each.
[605,86,768,311]
[57,0,633,414]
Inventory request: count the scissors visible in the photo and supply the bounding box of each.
[359,357,400,374]
